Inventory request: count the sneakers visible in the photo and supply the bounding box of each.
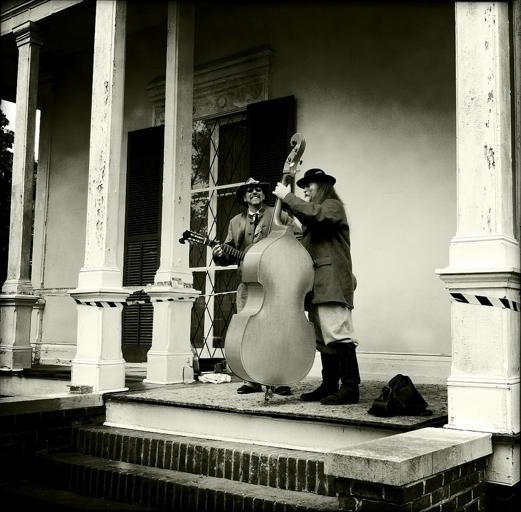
[274,386,291,395]
[238,386,262,392]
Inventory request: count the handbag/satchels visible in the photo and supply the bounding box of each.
[369,374,427,417]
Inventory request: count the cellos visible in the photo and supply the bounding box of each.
[226,133,316,387]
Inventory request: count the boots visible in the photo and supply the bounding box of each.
[300,344,340,401]
[320,342,359,405]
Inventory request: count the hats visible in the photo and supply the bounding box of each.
[236,177,271,208]
[297,169,336,189]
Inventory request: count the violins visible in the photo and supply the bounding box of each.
[179,230,257,273]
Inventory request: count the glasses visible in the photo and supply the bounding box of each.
[245,187,262,192]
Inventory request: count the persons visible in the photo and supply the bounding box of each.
[210,176,304,397]
[270,167,364,406]
